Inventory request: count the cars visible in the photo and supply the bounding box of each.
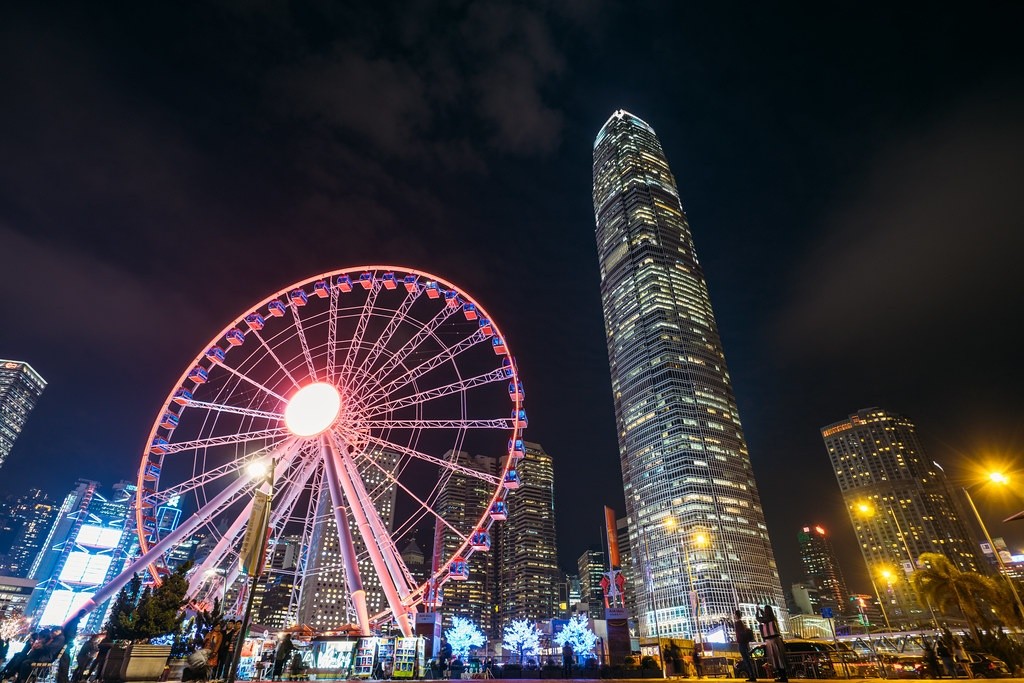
[851,652,924,679]
[915,650,1013,678]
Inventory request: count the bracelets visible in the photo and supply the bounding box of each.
[213,651,217,653]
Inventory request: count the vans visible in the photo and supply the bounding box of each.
[736,640,838,679]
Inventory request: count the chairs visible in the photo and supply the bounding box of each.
[425,659,434,679]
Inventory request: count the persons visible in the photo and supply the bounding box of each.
[440,636,493,679]
[661,641,703,679]
[755,605,788,682]
[735,609,756,682]
[218,620,243,682]
[209,620,236,683]
[0,627,117,683]
[203,622,223,680]
[922,636,973,680]
[272,634,299,682]
[562,641,573,679]
[262,650,277,679]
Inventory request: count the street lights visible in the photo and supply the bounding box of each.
[640,515,680,680]
[856,501,944,633]
[682,532,715,657]
[962,469,1024,627]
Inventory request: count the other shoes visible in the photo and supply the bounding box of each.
[746,679,756,682]
[775,676,788,682]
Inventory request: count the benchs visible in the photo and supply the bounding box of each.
[450,665,470,679]
[26,644,67,683]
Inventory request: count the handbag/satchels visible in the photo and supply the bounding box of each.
[759,620,779,638]
